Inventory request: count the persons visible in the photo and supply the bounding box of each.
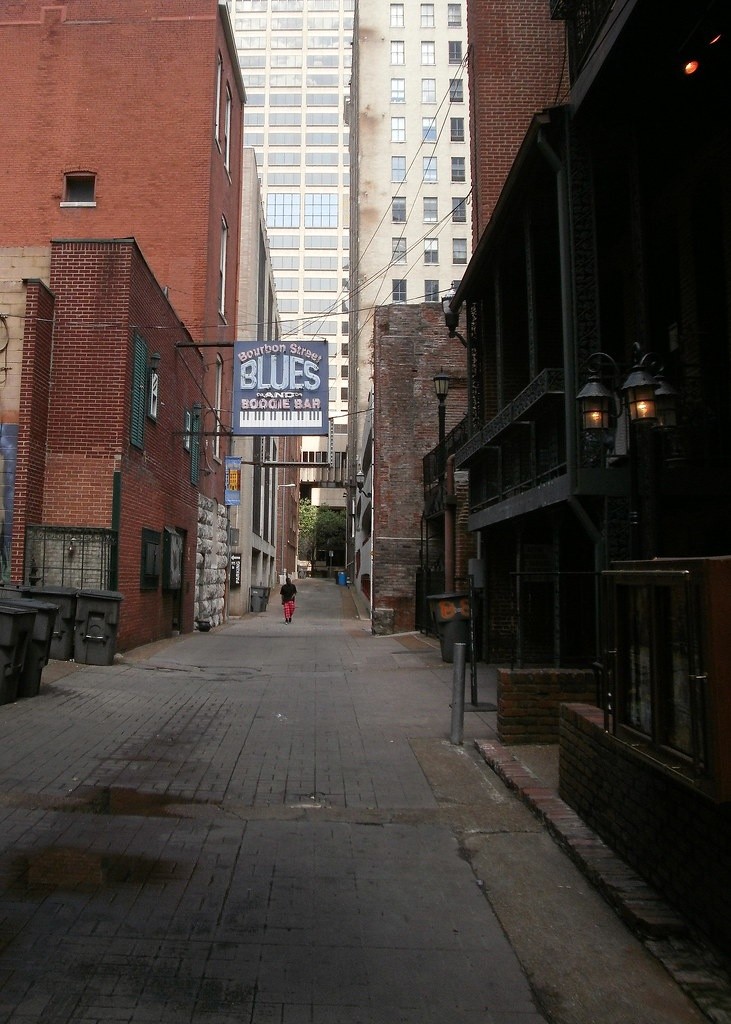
[280,578,297,624]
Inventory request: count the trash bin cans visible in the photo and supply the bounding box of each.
[29,585,81,661]
[251,586,272,612]
[74,589,126,666]
[427,593,473,663]
[334,569,347,585]
[0,597,61,706]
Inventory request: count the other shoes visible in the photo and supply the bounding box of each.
[285,619,288,624]
[289,618,291,623]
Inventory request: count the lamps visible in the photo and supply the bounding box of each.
[443,283,468,348]
[356,470,371,498]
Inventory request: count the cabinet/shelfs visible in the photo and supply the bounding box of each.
[601,555,731,804]
